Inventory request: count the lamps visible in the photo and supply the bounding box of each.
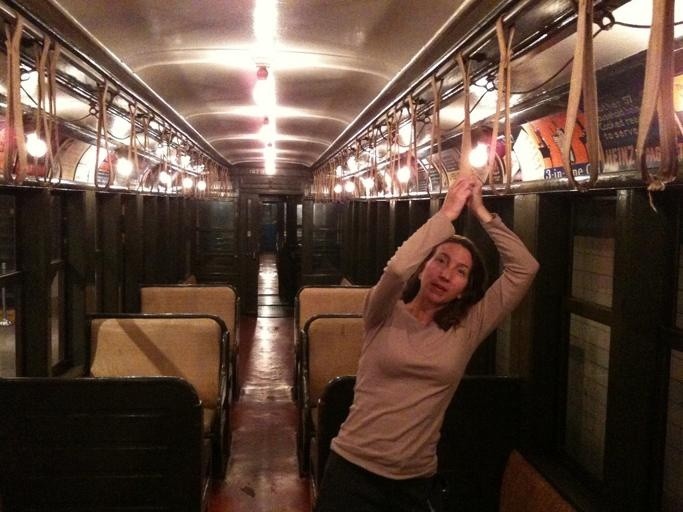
[113,153,134,176]
[295,286,377,330]
[23,124,48,158]
[467,135,491,168]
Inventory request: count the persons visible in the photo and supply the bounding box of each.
[313,173,540,511]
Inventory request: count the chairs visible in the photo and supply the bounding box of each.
[85,313,229,421]
[137,282,239,349]
[1,378,229,512]
[315,375,518,511]
[300,315,372,399]
